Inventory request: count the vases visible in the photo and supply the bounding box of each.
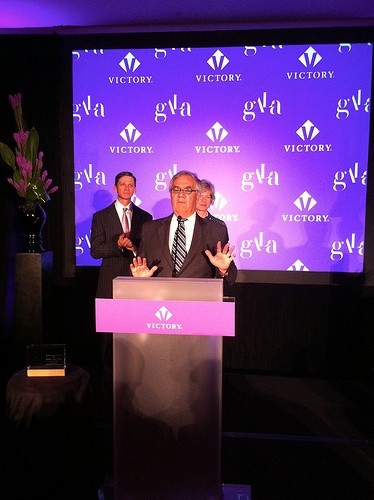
[17,203,46,253]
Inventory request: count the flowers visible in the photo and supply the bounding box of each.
[0,92,58,211]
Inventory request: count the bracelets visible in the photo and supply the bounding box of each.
[118,244,122,249]
[219,270,228,275]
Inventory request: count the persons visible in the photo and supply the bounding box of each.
[130,171,238,288]
[90,172,153,299]
[196,179,224,225]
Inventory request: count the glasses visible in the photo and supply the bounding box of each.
[169,186,199,195]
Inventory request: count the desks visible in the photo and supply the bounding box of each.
[5,363,93,500]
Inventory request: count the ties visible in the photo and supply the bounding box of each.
[121,208,130,239]
[171,217,188,275]
